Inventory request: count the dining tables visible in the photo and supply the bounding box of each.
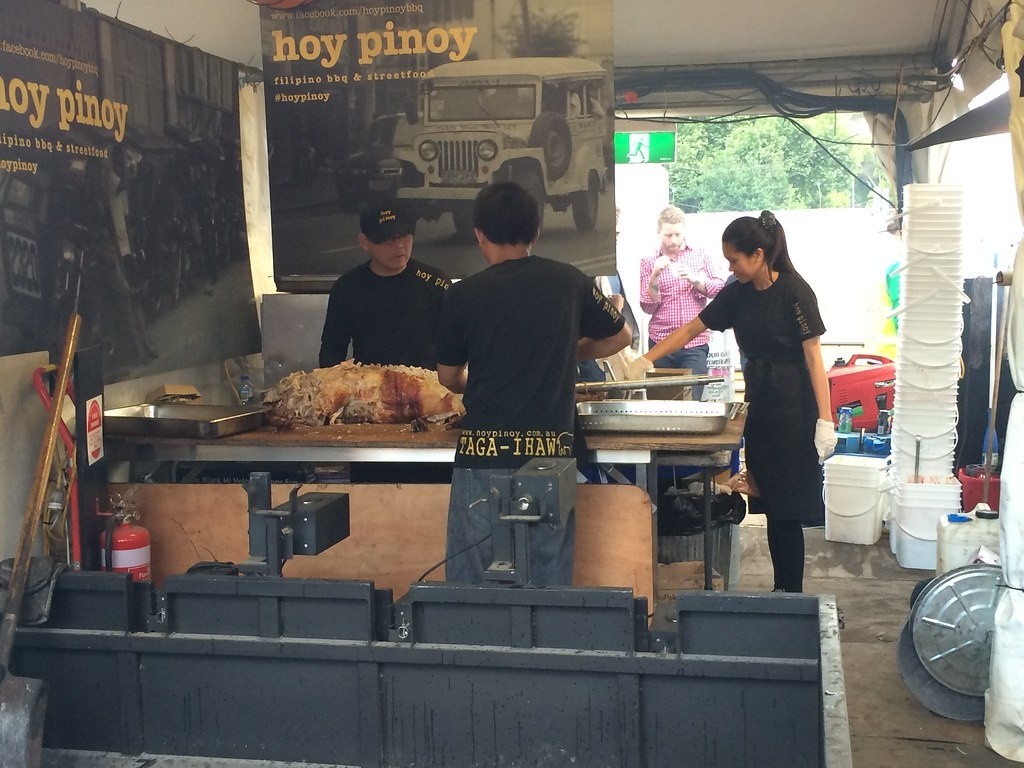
[103,396,750,589]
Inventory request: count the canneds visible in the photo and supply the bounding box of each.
[836,406,854,434]
[877,409,890,434]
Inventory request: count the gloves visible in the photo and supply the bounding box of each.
[814,419,838,465]
[621,357,653,380]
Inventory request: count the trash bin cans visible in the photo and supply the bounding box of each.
[651,485,746,588]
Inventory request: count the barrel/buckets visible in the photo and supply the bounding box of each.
[823,454,890,544]
[887,181,975,570]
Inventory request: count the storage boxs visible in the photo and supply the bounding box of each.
[821,182,1000,580]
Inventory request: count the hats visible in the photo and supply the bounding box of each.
[360,191,417,244]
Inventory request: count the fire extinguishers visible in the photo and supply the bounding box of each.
[94,493,152,586]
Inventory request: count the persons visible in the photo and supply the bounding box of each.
[436,182,634,592]
[626,210,841,596]
[83,119,163,366]
[639,205,726,403]
[319,195,454,372]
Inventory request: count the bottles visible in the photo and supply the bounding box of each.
[838,406,852,433]
[876,409,890,436]
[235,374,255,406]
[937,508,1001,582]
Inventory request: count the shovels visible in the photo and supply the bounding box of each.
[0,314,85,768]
